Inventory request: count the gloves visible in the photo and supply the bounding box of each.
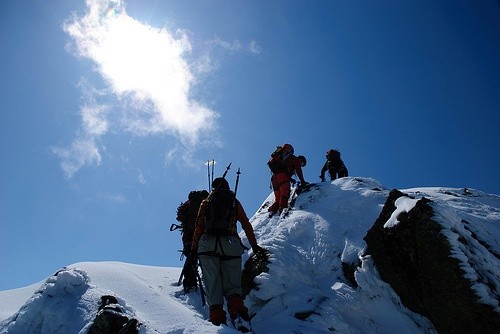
[189,250,198,261]
[252,245,263,254]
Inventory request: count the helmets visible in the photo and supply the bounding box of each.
[299,155,306,167]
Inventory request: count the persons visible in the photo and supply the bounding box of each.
[268,155,309,218]
[319,159,348,182]
[186,177,263,334]
[176,189,210,293]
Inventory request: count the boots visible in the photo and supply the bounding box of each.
[226,295,255,334]
[208,304,228,327]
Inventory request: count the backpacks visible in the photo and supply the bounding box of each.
[266,143,296,176]
